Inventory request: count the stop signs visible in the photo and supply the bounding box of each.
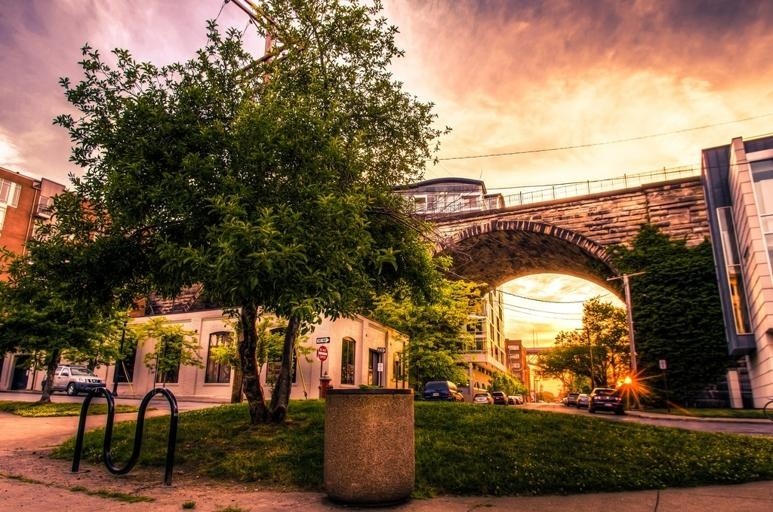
[317,346,328,361]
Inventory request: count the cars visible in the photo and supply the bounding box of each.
[492,391,524,405]
[42,366,107,397]
[473,393,494,404]
[588,388,625,415]
[422,381,465,403]
[563,393,589,409]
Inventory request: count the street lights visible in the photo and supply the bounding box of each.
[112,313,130,396]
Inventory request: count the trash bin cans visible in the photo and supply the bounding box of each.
[324,388,414,506]
